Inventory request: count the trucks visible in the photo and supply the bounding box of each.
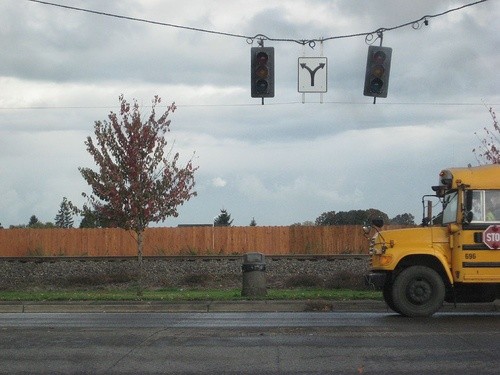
[363,163,500,317]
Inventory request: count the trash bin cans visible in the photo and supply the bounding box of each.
[241,252,267,296]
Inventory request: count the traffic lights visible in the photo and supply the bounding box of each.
[251,47,274,98]
[363,46,393,98]
